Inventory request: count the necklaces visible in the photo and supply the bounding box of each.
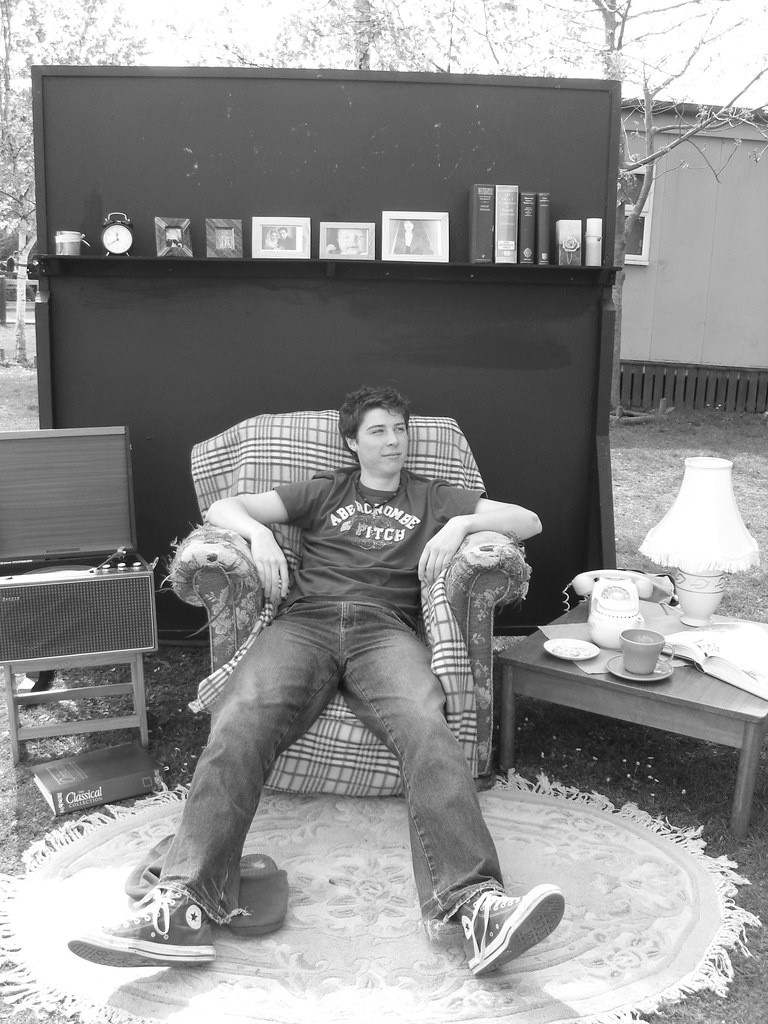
[356,471,402,519]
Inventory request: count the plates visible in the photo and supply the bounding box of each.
[606,654,674,681]
[543,638,600,661]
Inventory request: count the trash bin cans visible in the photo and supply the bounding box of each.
[0,275,7,326]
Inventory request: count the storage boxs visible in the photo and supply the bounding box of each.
[0,423,158,660]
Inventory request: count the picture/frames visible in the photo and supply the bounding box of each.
[251,215,310,257]
[205,217,245,257]
[155,215,194,257]
[319,221,377,259]
[382,211,454,260]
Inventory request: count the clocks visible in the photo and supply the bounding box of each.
[102,211,135,254]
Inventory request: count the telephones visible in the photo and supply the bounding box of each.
[572,567,672,649]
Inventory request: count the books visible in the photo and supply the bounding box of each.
[660,621,768,700]
[467,183,551,266]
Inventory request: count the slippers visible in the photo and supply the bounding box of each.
[125,834,177,897]
[225,854,289,934]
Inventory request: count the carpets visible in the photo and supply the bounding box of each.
[0,764,762,1024]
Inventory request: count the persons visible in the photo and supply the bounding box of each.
[27,255,39,293]
[327,228,367,255]
[394,220,432,255]
[265,228,296,250]
[5,251,17,278]
[66,380,565,976]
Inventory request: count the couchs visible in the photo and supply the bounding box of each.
[167,410,532,794]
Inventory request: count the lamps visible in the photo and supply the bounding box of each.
[637,457,760,625]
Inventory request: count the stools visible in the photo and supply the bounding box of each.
[0,653,151,762]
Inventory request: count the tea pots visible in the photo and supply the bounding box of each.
[587,592,645,651]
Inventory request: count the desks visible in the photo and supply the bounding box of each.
[497,597,768,843]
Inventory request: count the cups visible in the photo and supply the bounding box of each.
[619,628,675,675]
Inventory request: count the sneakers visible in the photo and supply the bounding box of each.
[461,884,565,976]
[67,887,216,967]
